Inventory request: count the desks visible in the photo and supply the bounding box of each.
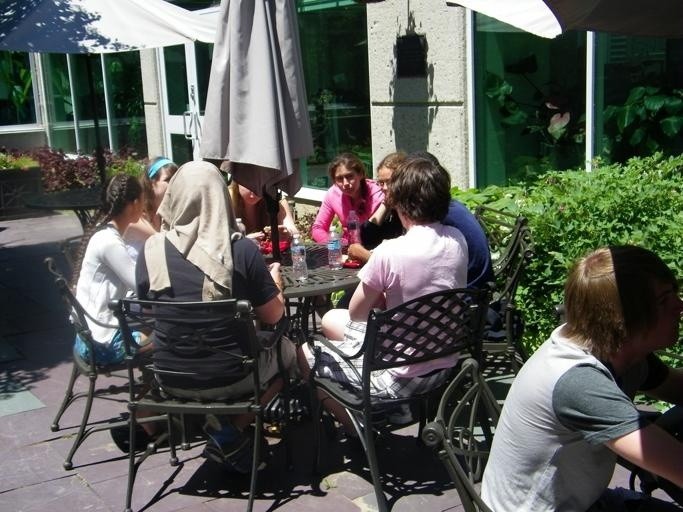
[25,188,106,234]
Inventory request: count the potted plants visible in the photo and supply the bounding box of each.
[0,151,41,215]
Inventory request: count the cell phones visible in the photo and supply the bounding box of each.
[341,259,362,268]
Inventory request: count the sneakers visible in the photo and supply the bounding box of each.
[107,411,154,454]
[201,413,267,473]
[340,401,412,445]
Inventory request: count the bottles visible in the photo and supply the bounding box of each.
[346,210,360,245]
[326,225,343,271]
[236,218,246,239]
[289,233,310,279]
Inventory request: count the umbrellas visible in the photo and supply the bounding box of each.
[198,0,316,264]
[0,0,220,185]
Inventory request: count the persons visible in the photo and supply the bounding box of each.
[67,151,496,476]
[478,244,683,511]
